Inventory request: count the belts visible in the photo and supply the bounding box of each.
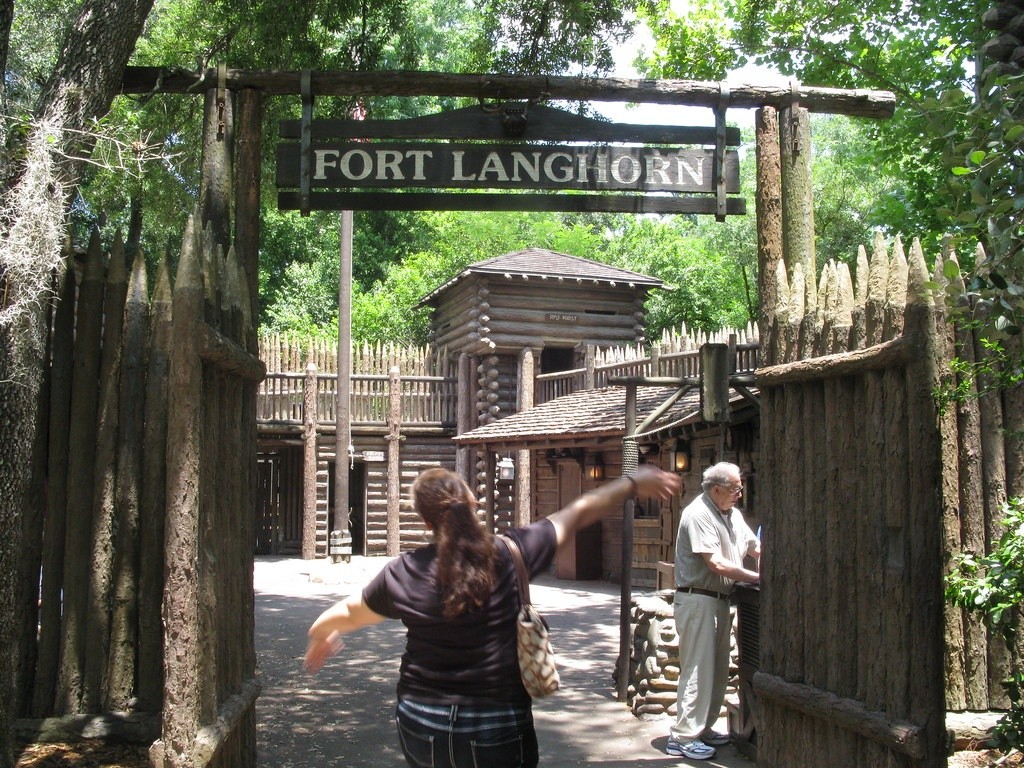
[677,587,731,600]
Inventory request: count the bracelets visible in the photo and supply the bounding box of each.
[624,473,638,500]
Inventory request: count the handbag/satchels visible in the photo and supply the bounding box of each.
[495,533,560,698]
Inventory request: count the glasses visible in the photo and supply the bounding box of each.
[727,487,744,496]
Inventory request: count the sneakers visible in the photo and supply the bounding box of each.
[700,729,730,744]
[666,737,716,760]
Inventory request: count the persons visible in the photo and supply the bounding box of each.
[302,467,684,768]
[665,462,760,761]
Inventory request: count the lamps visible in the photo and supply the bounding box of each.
[665,437,691,473]
[497,457,514,479]
[587,455,605,481]
